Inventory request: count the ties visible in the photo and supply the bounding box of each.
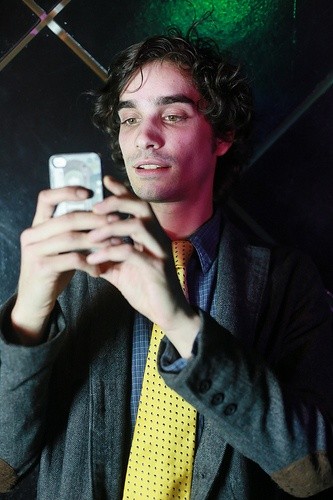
[122,240,198,499]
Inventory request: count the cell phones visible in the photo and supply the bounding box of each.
[49,153,106,256]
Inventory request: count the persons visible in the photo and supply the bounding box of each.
[0,29,333,499]
